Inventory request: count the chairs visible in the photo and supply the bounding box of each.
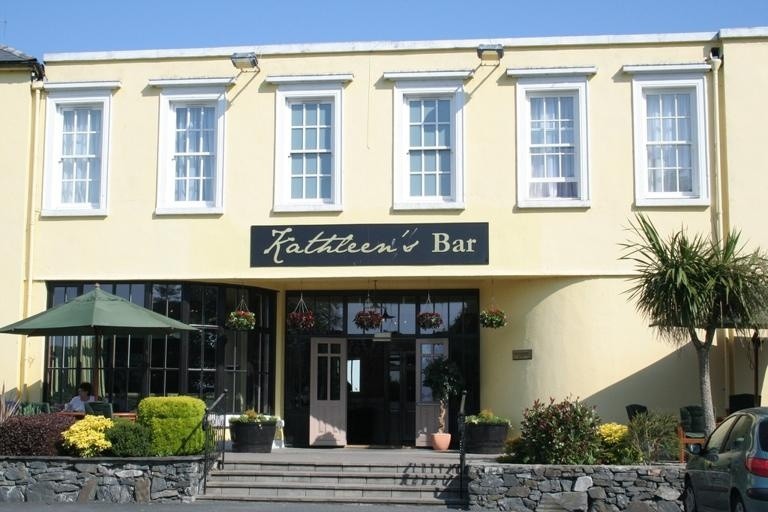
[724,393,760,418]
[624,402,649,422]
[23,400,139,422]
[676,404,710,462]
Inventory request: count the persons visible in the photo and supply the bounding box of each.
[69,382,95,413]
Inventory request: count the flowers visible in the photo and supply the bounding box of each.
[222,285,513,336]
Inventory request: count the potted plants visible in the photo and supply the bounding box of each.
[461,407,512,454]
[227,407,281,452]
[421,353,462,452]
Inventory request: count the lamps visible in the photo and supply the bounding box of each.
[475,42,505,68]
[229,50,261,74]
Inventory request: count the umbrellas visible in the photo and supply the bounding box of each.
[0,283,202,400]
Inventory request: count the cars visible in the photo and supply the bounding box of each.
[682,404,766,512]
